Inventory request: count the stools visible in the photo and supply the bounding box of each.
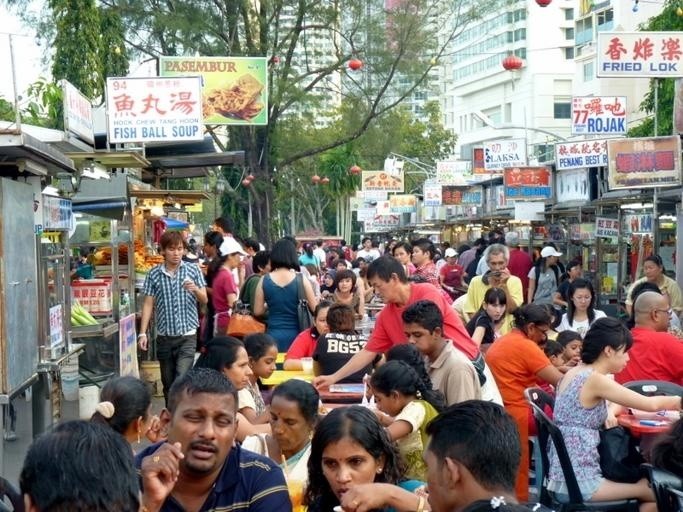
[526,436,544,500]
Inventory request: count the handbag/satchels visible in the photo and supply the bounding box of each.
[473,314,495,357]
[596,424,647,484]
[295,272,314,331]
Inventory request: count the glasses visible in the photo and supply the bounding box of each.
[572,295,592,303]
[656,309,673,316]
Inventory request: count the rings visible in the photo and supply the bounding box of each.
[352,500,362,507]
[152,455,161,464]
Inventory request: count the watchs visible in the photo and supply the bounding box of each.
[135,333,147,337]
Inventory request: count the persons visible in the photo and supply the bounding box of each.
[311,239,327,271]
[534,339,569,427]
[631,282,683,337]
[616,292,681,395]
[330,271,363,317]
[312,304,382,385]
[551,260,583,307]
[297,241,319,269]
[538,304,562,341]
[373,241,382,254]
[202,232,224,338]
[505,229,533,298]
[392,241,414,275]
[439,242,447,254]
[458,238,484,277]
[422,400,557,512]
[240,254,275,307]
[358,238,378,261]
[130,367,293,511]
[215,218,245,251]
[319,273,335,299]
[254,238,319,348]
[351,260,360,275]
[411,236,440,288]
[489,230,501,238]
[558,279,608,340]
[74,246,91,265]
[625,255,682,323]
[364,344,445,414]
[463,288,507,356]
[185,337,276,445]
[284,302,335,370]
[207,238,250,342]
[476,237,503,272]
[547,316,683,511]
[528,247,564,303]
[139,232,207,417]
[300,405,430,512]
[485,302,561,435]
[370,361,440,481]
[324,245,352,268]
[341,240,353,260]
[441,246,468,296]
[357,258,367,268]
[401,302,482,405]
[89,376,162,454]
[334,261,346,272]
[311,256,503,403]
[233,332,276,417]
[241,379,326,512]
[553,329,584,369]
[462,245,487,283]
[384,239,395,257]
[19,421,143,511]
[467,244,523,333]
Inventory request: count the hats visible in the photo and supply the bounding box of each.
[539,245,564,259]
[444,247,459,259]
[219,238,249,258]
[325,270,337,281]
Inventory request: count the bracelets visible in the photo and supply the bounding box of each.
[417,496,426,512]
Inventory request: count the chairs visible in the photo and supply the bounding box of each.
[620,380,683,400]
[639,464,683,512]
[523,386,638,512]
